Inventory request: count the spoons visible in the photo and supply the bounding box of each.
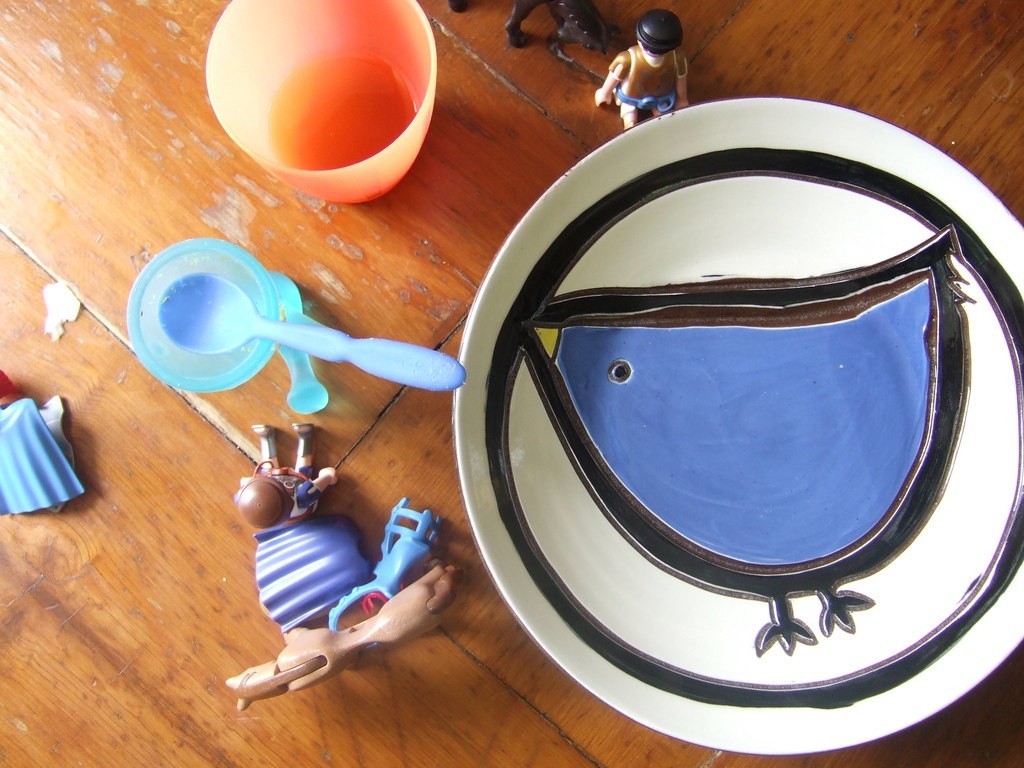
[160,272,465,396]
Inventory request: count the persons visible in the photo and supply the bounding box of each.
[234,420,337,532]
[595,8,689,131]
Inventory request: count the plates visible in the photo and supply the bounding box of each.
[452,96,1024,754]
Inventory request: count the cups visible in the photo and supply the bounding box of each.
[207,1,439,208]
[126,239,266,395]
